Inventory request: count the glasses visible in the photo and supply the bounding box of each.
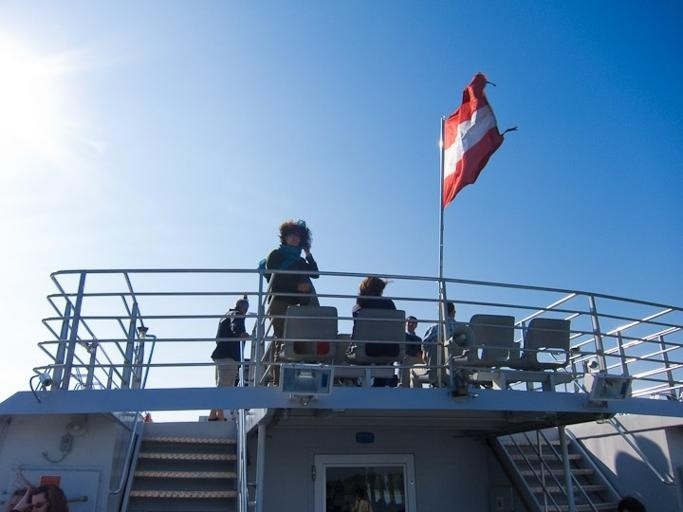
[30,500,48,509]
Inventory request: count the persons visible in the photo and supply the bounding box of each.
[264,218,319,389]
[409,301,469,388]
[617,494,648,512]
[398,314,423,388]
[29,485,68,512]
[349,488,373,512]
[206,297,249,423]
[351,274,400,388]
[6,487,31,511]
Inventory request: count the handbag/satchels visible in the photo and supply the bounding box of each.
[343,341,368,364]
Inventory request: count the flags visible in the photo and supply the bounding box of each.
[440,70,519,208]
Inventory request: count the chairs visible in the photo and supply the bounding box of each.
[261,304,576,393]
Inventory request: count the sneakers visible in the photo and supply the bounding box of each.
[207,417,228,422]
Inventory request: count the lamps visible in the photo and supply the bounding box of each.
[581,369,638,403]
